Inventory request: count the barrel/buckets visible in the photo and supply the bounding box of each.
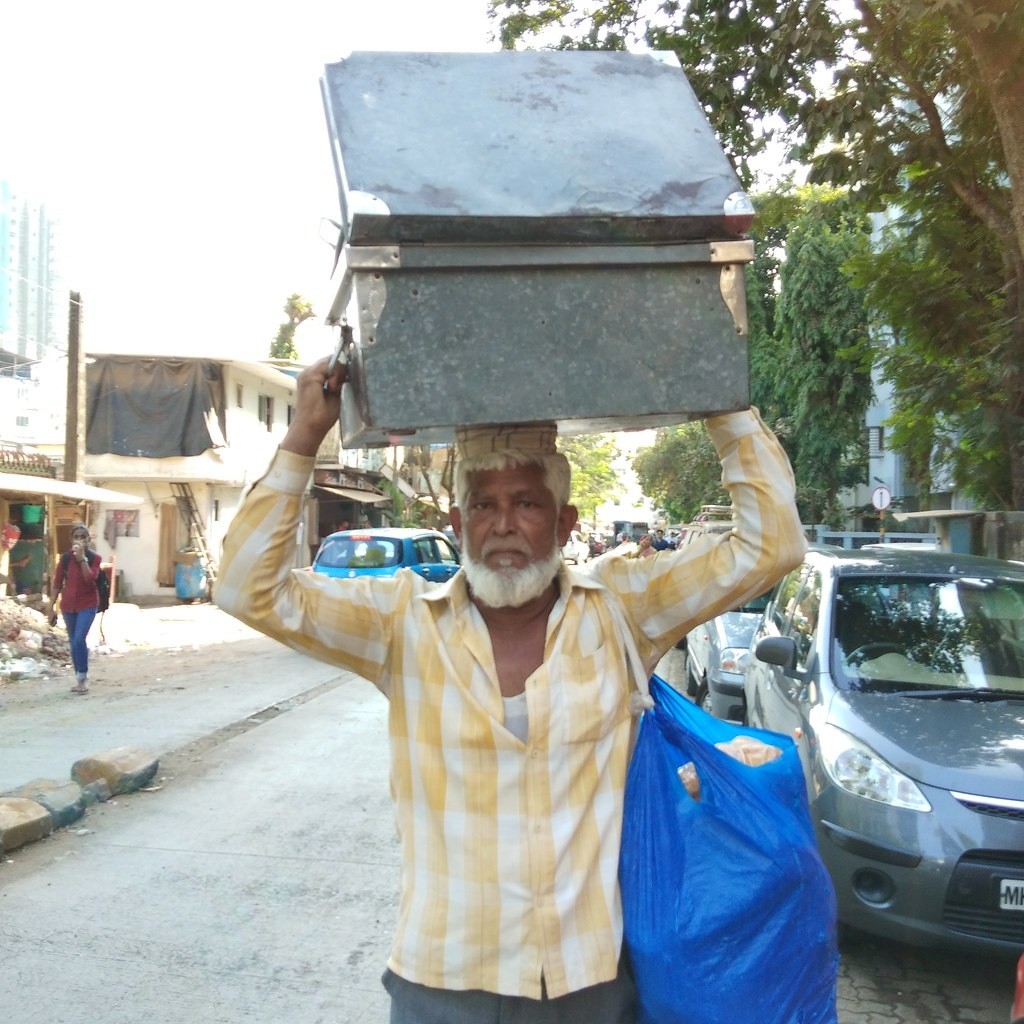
[175,551,206,599]
[21,504,42,524]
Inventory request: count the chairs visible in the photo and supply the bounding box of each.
[838,600,907,667]
[930,602,1009,677]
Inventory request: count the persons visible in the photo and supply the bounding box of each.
[210,346,810,1024]
[587,532,596,559]
[46,526,102,693]
[336,520,349,531]
[363,520,373,528]
[637,529,677,558]
[615,528,623,542]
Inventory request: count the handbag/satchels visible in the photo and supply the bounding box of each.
[617,671,841,1023]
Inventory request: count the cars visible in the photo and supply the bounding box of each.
[589,531,607,555]
[560,530,590,565]
[311,528,460,587]
[742,545,1024,960]
[686,542,846,726]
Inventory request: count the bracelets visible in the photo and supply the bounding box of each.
[77,557,89,562]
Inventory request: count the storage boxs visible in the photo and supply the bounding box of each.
[321,47,759,439]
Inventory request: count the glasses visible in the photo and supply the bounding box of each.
[74,534,86,540]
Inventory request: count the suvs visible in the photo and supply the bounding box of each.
[674,503,733,549]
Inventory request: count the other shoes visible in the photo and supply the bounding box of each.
[71,685,88,695]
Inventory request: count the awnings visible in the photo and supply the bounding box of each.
[314,485,392,503]
[0,473,144,505]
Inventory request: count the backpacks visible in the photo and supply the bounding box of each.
[62,550,110,614]
